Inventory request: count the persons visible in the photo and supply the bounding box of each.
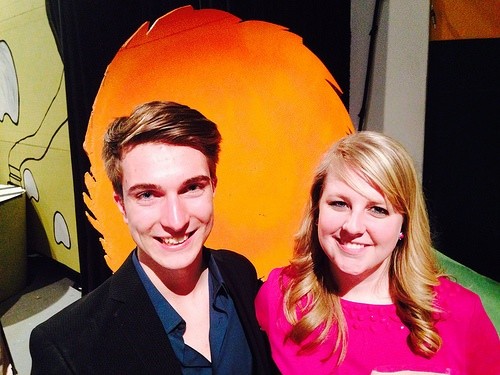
[29,101,279,375]
[253,130,500,375]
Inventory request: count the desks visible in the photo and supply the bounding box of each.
[0,183,26,304]
